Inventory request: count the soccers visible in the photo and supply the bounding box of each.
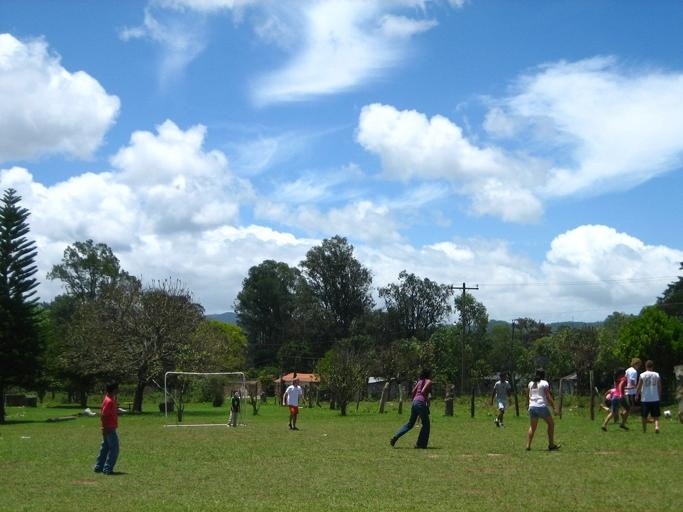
[664,410,671,418]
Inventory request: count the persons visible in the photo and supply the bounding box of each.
[490,371,512,428]
[525,367,561,451]
[92,379,120,475]
[390,368,433,449]
[226,390,241,427]
[599,358,664,433]
[282,377,308,430]
